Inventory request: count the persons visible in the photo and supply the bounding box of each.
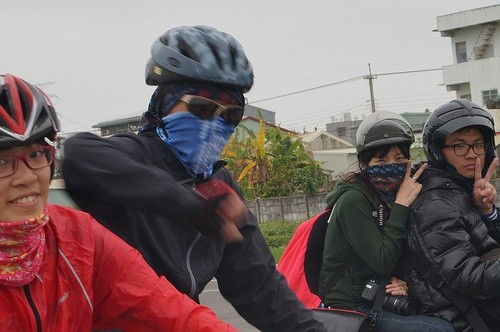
[0,73,238,332]
[62,24,328,332]
[278,109,455,332]
[405,99,500,332]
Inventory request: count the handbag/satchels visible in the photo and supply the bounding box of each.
[303,307,375,331]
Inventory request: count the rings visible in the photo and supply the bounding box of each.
[413,176,418,180]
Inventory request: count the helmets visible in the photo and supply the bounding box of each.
[422,98,495,163]
[145,24,253,93]
[354,108,415,159]
[0,72,60,147]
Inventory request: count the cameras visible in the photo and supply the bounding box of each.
[361,280,416,316]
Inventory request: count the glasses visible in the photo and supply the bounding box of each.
[439,143,488,156]
[180,92,243,135]
[0,146,56,179]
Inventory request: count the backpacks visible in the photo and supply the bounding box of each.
[276,188,386,311]
